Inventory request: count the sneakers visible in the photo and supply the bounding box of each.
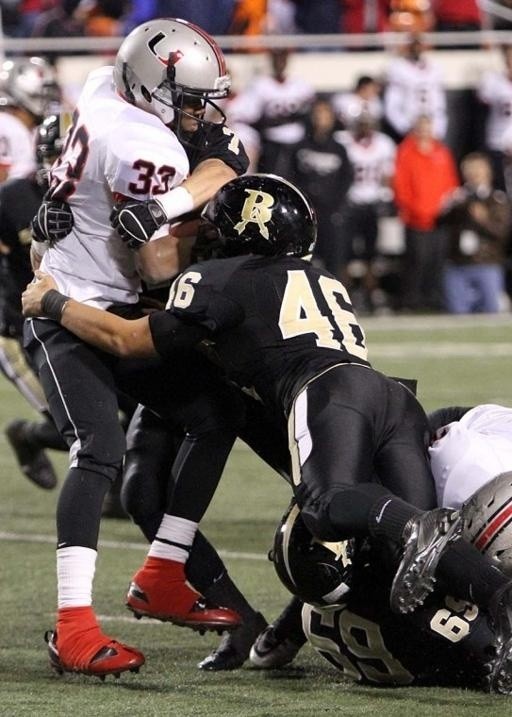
[251,607,306,670]
[196,613,267,671]
[6,419,59,492]
[124,575,238,633]
[47,630,149,678]
[386,506,460,615]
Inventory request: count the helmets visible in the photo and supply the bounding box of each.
[269,498,361,617]
[2,57,63,117]
[197,171,321,271]
[115,18,233,148]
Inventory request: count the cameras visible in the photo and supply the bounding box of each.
[463,178,495,205]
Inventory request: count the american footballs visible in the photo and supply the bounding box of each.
[170,219,207,237]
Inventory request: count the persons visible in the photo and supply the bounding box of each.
[4,17,512,697]
[1,0,511,338]
